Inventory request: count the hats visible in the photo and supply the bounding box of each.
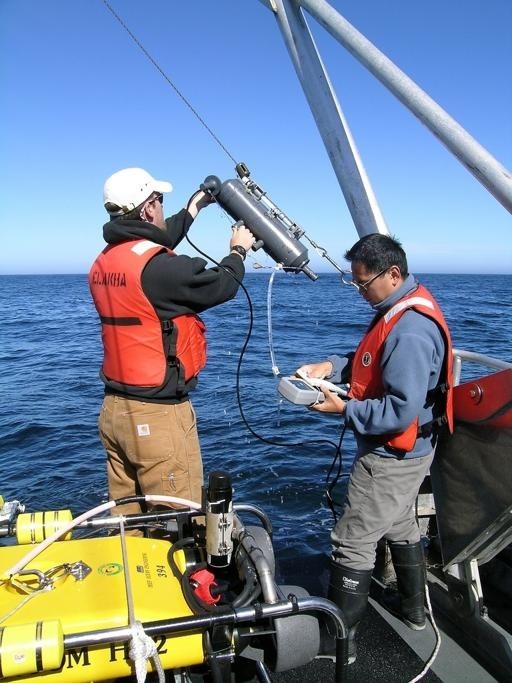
[102,166,174,218]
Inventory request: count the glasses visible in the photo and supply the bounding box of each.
[350,266,391,292]
[148,192,164,206]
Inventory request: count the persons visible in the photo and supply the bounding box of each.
[87,167,255,535]
[306,234,454,665]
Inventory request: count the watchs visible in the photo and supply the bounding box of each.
[231,246,247,261]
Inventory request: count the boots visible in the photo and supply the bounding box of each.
[312,554,376,667]
[380,539,428,632]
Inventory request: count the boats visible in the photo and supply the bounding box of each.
[0,0,511,681]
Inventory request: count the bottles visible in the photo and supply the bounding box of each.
[206,469,234,567]
